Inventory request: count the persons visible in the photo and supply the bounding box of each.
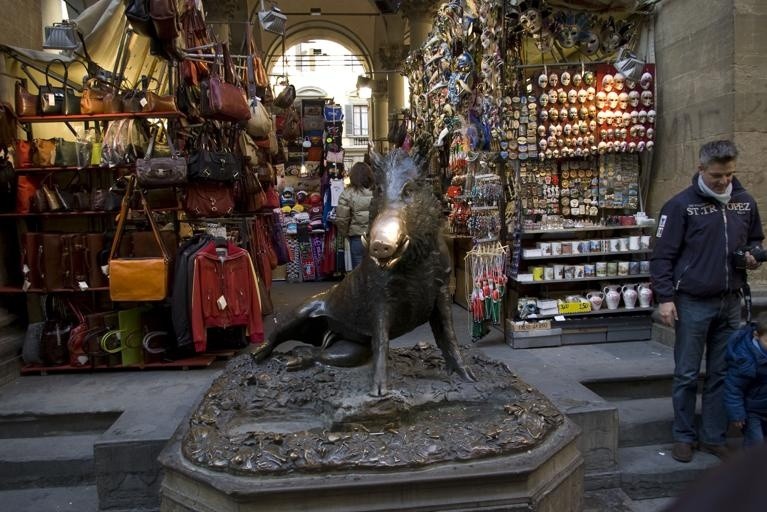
[721,310,767,451]
[336,162,374,270]
[650,139,766,462]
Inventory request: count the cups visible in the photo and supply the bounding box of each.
[531,234,654,281]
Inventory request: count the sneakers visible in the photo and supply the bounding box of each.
[698,439,737,464]
[671,441,692,462]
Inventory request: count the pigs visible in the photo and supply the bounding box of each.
[248,139,481,398]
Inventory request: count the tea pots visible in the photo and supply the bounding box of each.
[586,282,653,311]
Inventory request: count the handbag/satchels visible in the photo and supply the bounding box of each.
[1,159,15,213]
[20,230,178,302]
[14,80,178,116]
[16,138,190,212]
[1,101,17,148]
[281,105,344,178]
[254,215,292,315]
[21,303,200,370]
[124,1,186,66]
[186,60,280,216]
[274,84,297,109]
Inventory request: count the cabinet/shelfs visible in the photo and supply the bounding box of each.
[3,109,236,372]
[503,214,656,351]
